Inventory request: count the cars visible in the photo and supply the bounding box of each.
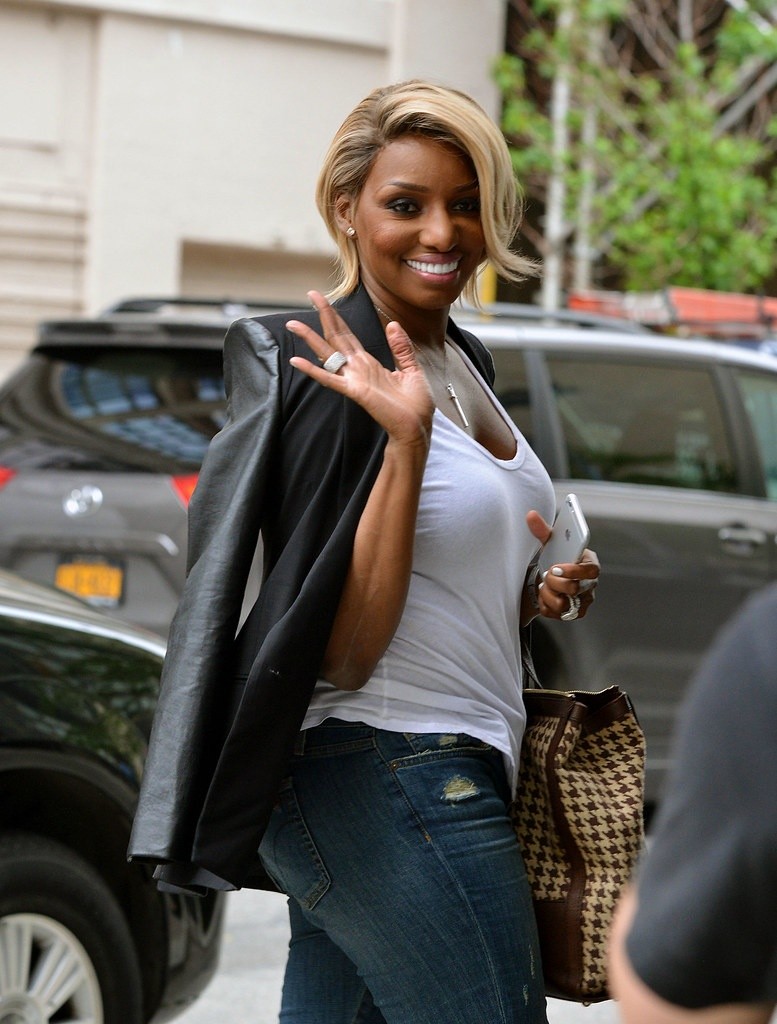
[1,569,226,1023]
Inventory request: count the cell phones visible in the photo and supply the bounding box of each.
[537,494,590,579]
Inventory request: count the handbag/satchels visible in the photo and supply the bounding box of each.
[509,558,649,1005]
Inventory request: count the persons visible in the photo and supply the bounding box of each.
[118,78,601,1024]
[606,582,777,1024]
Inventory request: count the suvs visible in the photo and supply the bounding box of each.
[0,294,775,817]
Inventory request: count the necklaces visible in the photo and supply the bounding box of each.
[371,306,470,428]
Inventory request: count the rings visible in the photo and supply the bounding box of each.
[321,352,350,376]
[558,595,581,623]
